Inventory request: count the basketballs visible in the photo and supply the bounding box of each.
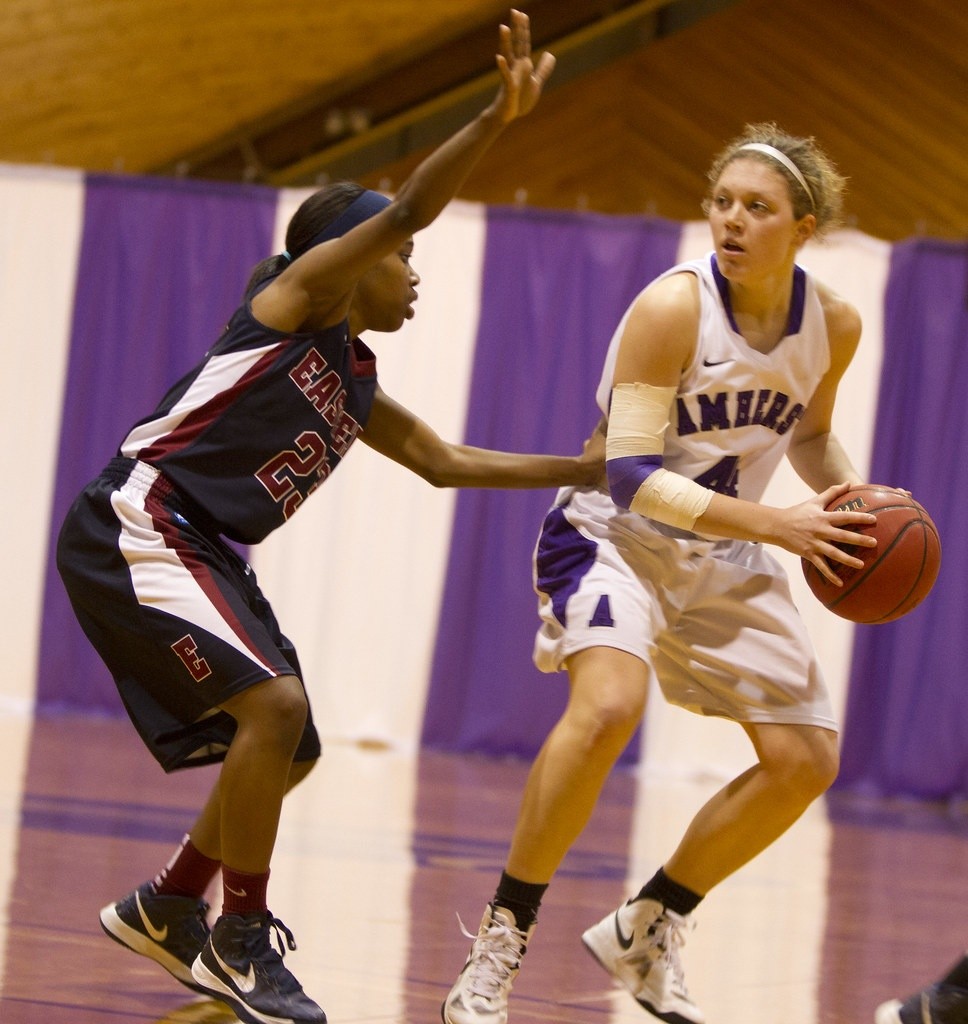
[800,484,942,626]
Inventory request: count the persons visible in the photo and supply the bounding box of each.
[55,9,610,1023]
[874,955,968,1024]
[441,119,916,1024]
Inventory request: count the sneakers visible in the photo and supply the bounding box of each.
[190,910,327,1024]
[99,881,214,995]
[580,898,704,1024]
[441,902,538,1024]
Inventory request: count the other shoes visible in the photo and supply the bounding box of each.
[873,981,968,1024]
